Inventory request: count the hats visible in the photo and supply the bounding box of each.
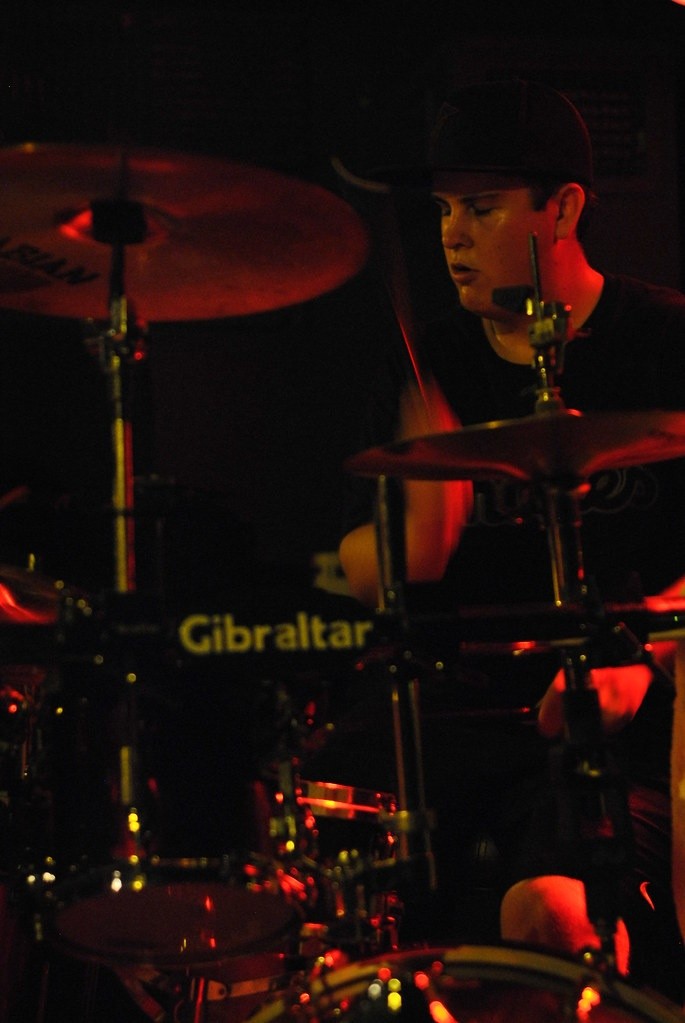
[367,81,596,185]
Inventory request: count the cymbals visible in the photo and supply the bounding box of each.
[343,413,685,497]
[0,140,362,320]
[2,562,83,627]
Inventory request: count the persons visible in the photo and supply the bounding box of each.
[334,66,684,971]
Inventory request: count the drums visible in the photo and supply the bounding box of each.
[55,878,683,1023]
